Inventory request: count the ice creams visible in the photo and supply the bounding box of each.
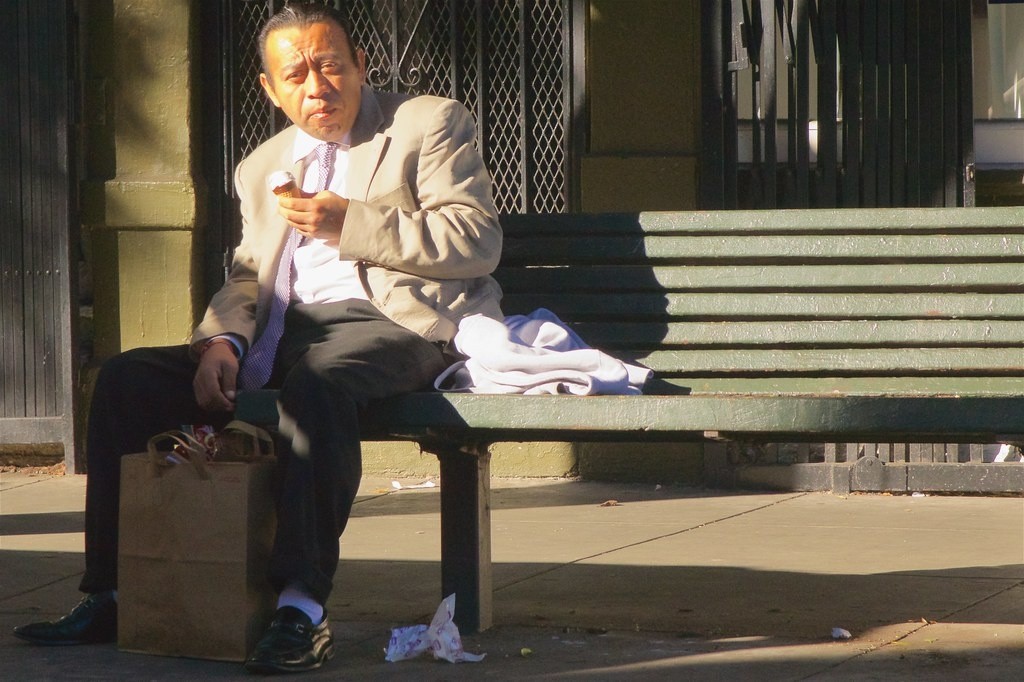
[268,170,301,198]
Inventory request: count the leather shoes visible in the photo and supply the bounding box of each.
[11,592,117,645]
[244,603,335,672]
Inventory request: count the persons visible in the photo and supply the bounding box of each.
[13,1,503,674]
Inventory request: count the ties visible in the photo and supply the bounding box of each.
[239,142,338,397]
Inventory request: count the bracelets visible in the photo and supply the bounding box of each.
[202,339,237,354]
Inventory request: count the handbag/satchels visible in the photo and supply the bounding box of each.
[117,422,283,663]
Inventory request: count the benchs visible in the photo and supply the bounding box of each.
[234,204,1024,632]
